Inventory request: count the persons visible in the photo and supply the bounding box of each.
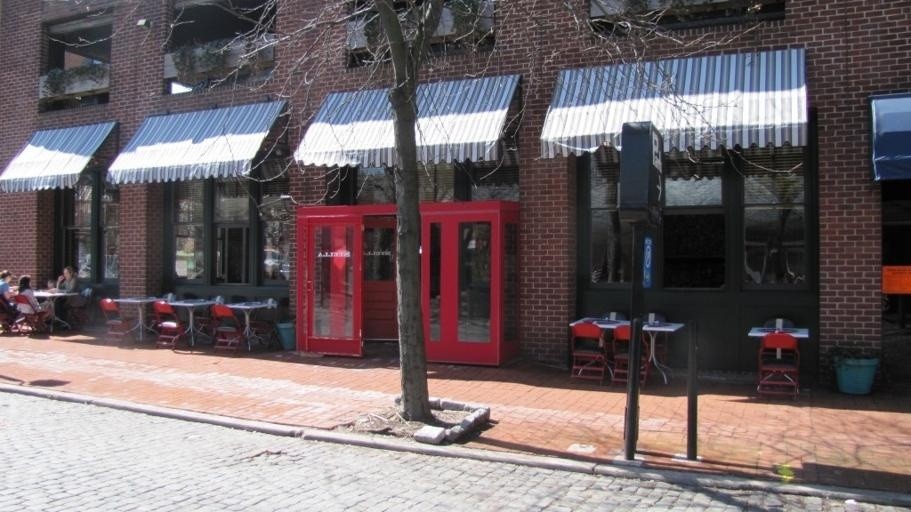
[16,274,55,326]
[51,265,83,329]
[0,270,18,334]
[40,279,56,292]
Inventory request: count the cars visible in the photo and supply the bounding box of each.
[263,247,284,275]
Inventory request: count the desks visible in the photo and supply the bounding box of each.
[747,326,810,399]
[570,317,686,384]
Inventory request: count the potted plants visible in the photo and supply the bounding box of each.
[830,336,884,395]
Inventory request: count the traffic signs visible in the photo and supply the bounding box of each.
[648,126,667,210]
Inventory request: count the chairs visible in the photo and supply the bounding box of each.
[100,293,284,358]
[570,311,669,391]
[757,318,801,372]
[0,288,93,339]
[756,333,801,401]
